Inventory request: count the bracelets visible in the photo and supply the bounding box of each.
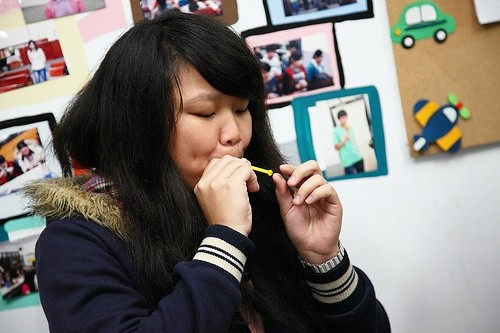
[297,238,345,274]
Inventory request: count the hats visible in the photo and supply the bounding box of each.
[17,139,28,151]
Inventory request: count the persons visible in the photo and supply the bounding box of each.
[0,156,23,184]
[26,40,48,83]
[43,0,84,20]
[25,7,392,333]
[15,139,46,173]
[332,109,366,174]
[253,41,334,99]
[137,0,219,20]
